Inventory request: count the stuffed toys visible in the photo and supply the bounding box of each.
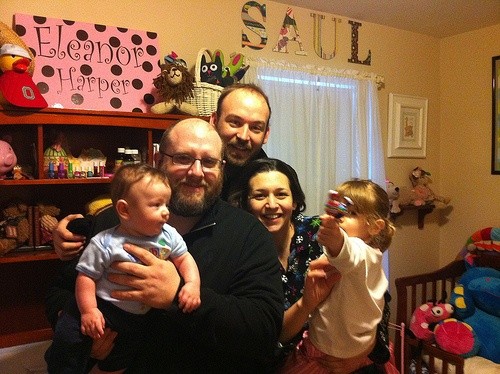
[0,20,50,110]
[387,167,451,213]
[410,227,500,365]
[0,201,60,256]
[150,49,250,114]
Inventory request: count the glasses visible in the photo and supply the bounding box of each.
[159,151,227,170]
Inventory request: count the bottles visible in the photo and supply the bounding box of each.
[113,147,140,174]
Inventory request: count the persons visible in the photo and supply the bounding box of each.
[53,118,284,374]
[212,83,271,208]
[242,158,392,362]
[45,163,201,374]
[282,178,400,374]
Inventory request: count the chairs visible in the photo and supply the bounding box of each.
[395,227,500,374]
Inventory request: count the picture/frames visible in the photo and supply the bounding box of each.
[387,93,428,158]
[491,55,500,175]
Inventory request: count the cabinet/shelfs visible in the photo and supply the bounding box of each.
[0,105,213,348]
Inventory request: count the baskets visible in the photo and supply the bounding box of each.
[184,48,225,117]
